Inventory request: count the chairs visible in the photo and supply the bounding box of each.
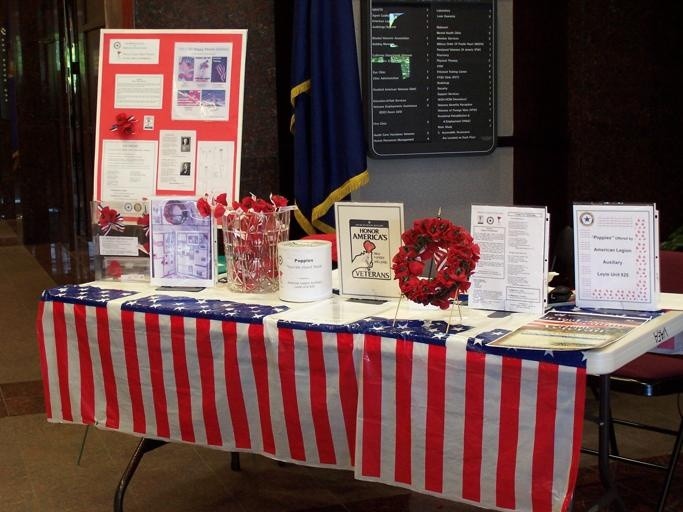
[587,251,683,512]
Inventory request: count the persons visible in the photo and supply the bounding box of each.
[181,137,190,152]
[199,60,209,78]
[206,93,216,103]
[6,61,22,174]
[189,91,198,104]
[181,162,189,175]
[179,56,194,82]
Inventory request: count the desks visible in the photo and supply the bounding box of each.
[37,272,683,512]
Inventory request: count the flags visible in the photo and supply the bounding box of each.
[289,0,368,241]
[216,58,226,82]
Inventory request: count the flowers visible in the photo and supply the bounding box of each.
[390,218,480,310]
[98,204,125,236]
[197,193,288,292]
[106,261,123,280]
[109,113,137,136]
[137,212,149,236]
